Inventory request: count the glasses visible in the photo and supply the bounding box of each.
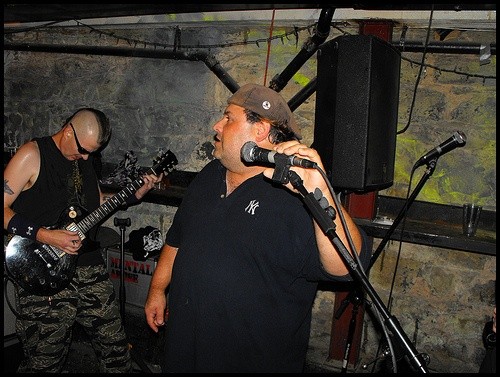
[69,122,99,158]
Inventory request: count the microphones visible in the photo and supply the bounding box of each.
[241,141,317,168]
[414,132,467,167]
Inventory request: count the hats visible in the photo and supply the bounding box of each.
[226,83,303,141]
[129,226,164,261]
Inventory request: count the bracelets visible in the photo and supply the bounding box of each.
[120,193,141,207]
[6,213,40,240]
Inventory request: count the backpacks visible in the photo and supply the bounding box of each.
[76,225,121,266]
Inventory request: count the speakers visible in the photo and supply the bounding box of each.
[314,33,402,192]
[3,277,20,341]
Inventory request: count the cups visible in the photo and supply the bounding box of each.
[8,147,20,158]
[462,202,482,237]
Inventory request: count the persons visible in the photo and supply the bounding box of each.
[4,108,162,373]
[145,84,369,373]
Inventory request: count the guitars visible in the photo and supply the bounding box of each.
[7,151,178,297]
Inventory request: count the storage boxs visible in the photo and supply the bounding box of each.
[106,244,161,308]
